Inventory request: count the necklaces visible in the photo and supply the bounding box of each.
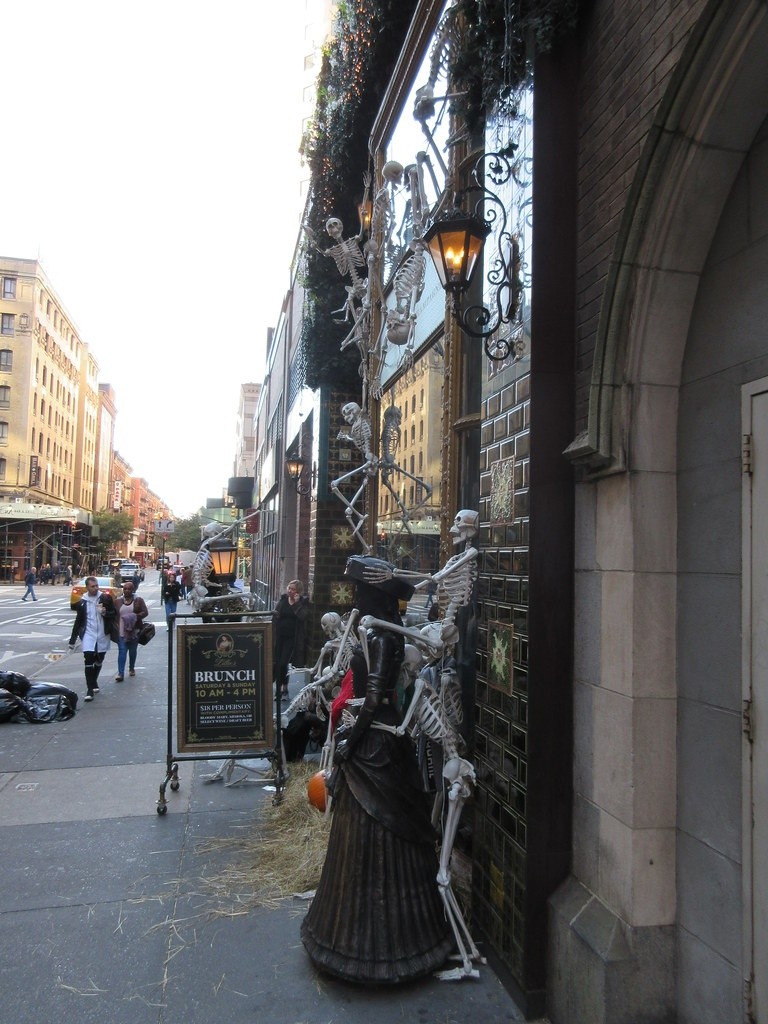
[125,598,131,603]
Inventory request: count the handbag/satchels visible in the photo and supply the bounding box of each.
[139,623,155,645]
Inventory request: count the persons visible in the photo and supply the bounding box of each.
[9,563,140,601]
[423,579,436,608]
[112,582,149,681]
[158,563,194,632]
[271,579,310,701]
[68,576,117,701]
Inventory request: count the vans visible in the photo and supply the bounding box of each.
[108,558,129,568]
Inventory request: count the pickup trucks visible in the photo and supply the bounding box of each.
[119,562,145,583]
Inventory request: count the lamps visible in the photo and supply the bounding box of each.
[422,143,519,362]
[286,443,318,502]
[205,531,239,582]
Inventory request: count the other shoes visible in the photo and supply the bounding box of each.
[22,597,27,601]
[33,599,38,601]
[116,675,124,681]
[93,688,100,693]
[282,691,289,700]
[273,697,276,701]
[166,624,169,632]
[84,695,94,700]
[129,671,135,676]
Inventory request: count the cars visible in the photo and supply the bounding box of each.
[157,559,169,571]
[71,576,124,611]
[172,565,186,573]
